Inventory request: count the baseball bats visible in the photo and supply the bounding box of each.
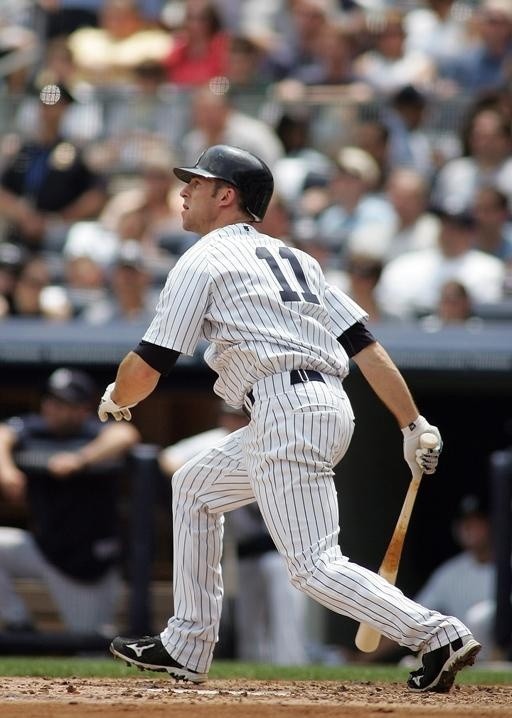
[355,432,439,654]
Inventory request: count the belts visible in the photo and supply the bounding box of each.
[241,370,326,420]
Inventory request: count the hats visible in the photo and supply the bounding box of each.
[31,363,101,407]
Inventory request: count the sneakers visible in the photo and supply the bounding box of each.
[406,634,485,696]
[108,631,211,688]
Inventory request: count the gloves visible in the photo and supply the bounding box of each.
[399,415,445,482]
[95,382,140,425]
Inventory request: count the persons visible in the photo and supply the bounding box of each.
[99,146,481,692]
[1,1,511,665]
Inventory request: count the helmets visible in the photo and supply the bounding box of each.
[170,144,276,224]
